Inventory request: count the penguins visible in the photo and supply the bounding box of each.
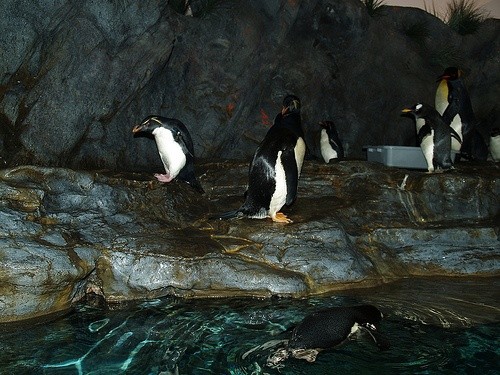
[273,304,383,362]
[433,66,489,166]
[132,115,206,195]
[401,102,462,175]
[318,120,345,164]
[207,94,308,224]
